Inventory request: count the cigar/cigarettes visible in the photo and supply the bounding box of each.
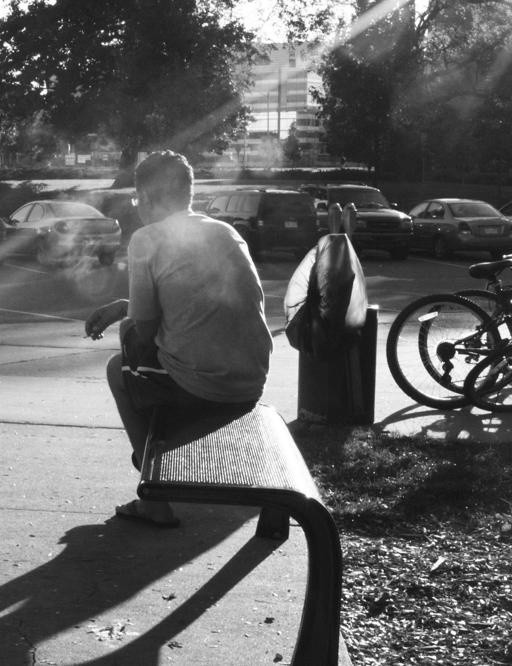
[82,336,90,339]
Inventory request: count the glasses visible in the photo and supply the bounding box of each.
[130,196,142,208]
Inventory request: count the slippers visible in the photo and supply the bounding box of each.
[131,450,142,473]
[115,499,180,529]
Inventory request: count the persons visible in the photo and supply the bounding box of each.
[80,150,275,531]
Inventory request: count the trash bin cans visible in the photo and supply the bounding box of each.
[65,153,77,165]
[298,304,377,426]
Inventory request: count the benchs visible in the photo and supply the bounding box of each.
[138,394,342,664]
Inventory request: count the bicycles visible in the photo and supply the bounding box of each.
[386,254,512,413]
